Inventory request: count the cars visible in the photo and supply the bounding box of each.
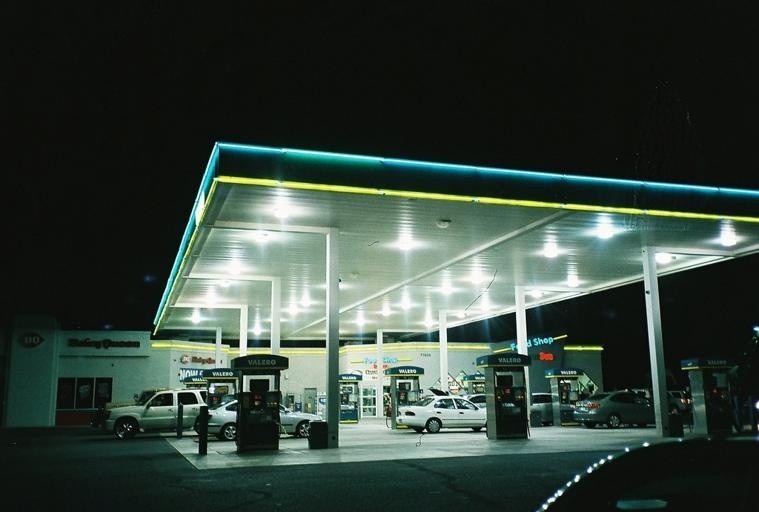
[396,394,487,432]
[668,390,690,414]
[208,399,322,440]
[574,390,654,428]
[532,393,575,423]
[104,388,209,440]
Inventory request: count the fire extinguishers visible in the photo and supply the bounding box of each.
[386,405,392,417]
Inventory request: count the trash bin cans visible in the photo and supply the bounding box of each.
[308,421,328,449]
[669,412,684,437]
